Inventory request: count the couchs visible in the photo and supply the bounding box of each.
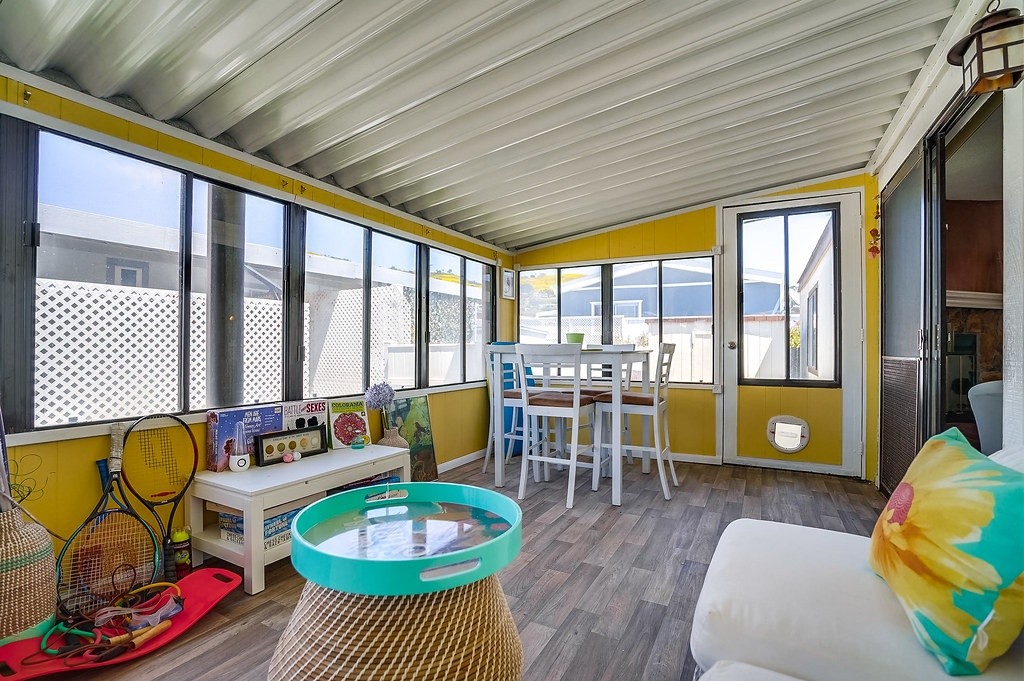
[690,379,1024,680]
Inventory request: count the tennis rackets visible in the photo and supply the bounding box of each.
[55,421,165,622]
[119,413,200,590]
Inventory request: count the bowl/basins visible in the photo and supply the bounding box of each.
[566,333,584,346]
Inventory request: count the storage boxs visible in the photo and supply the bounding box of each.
[206,489,327,549]
[207,400,372,472]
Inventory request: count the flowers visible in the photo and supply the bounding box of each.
[364,384,395,429]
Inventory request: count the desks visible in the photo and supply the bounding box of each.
[184,446,411,593]
[495,349,652,505]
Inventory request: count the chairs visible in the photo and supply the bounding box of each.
[484,340,679,509]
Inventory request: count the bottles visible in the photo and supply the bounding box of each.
[351,430,364,449]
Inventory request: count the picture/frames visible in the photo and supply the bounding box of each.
[379,394,439,482]
[500,269,516,300]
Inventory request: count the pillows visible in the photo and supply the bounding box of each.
[868,427,1024,674]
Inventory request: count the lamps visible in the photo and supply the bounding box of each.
[944,0,1024,96]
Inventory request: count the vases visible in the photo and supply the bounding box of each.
[377,428,410,449]
